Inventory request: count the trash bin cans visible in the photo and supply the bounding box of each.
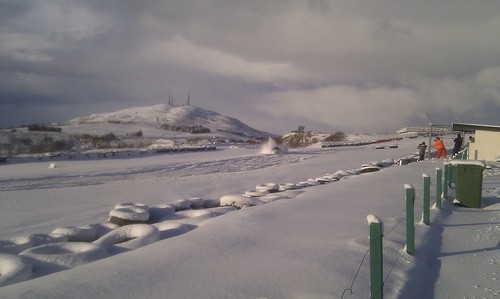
[452,160,485,208]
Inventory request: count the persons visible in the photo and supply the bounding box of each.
[434,137,446,159]
[469,135,474,143]
[451,134,463,160]
[417,141,428,160]
